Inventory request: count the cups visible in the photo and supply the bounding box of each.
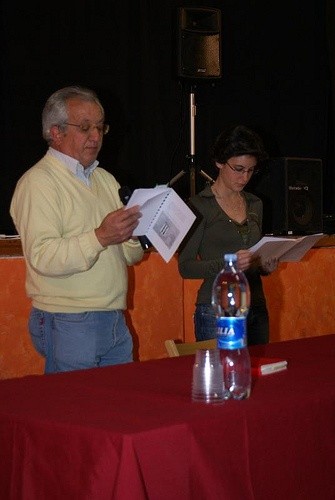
[192,350,225,403]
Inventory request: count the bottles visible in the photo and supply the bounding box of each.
[211,254,251,400]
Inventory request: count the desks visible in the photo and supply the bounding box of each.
[0,332,335,500]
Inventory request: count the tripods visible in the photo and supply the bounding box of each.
[166,82,216,196]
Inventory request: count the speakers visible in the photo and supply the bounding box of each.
[255,157,322,235]
[177,7,223,82]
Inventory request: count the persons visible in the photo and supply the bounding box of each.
[177,125,279,351]
[8,86,153,374]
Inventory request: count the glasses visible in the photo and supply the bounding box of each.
[64,121,109,134]
[227,161,259,176]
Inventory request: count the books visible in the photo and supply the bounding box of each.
[221,350,287,378]
[125,185,197,263]
[248,232,323,272]
